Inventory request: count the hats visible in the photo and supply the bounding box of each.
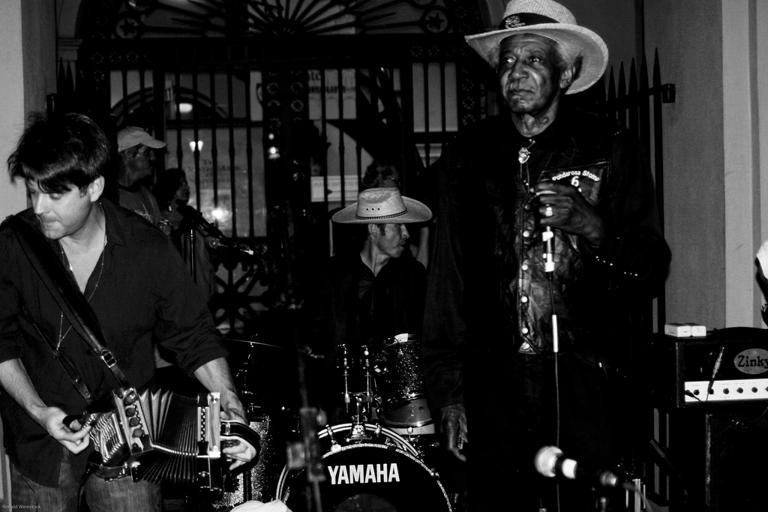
[462,0,609,95]
[331,186,433,225]
[117,126,168,153]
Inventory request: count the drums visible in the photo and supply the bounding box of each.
[276,421,451,510]
[335,332,437,437]
[222,341,293,419]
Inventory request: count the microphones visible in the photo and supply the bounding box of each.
[534,445,624,491]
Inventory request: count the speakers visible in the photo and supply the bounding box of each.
[669,405,768,512]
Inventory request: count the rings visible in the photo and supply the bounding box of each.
[544,206,553,218]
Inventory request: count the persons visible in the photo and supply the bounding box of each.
[316,185,435,353]
[413,1,672,510]
[155,167,212,298]
[113,125,169,224]
[363,148,432,275]
[1,110,260,511]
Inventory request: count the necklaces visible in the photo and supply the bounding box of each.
[40,236,106,360]
